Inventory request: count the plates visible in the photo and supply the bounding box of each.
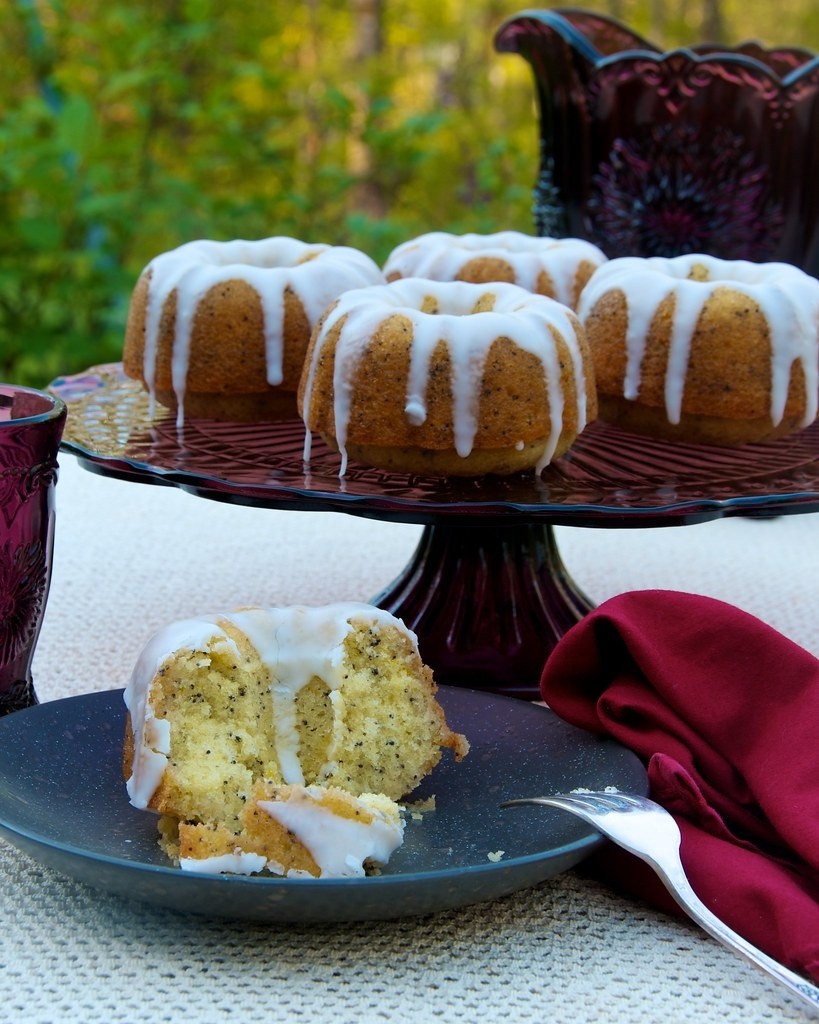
[0,685,652,923]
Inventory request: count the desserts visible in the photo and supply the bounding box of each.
[120,599,472,882]
[118,231,819,477]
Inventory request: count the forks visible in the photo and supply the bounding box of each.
[497,791,819,1012]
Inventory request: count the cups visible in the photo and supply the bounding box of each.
[494,9,819,278]
[0,384,67,717]
